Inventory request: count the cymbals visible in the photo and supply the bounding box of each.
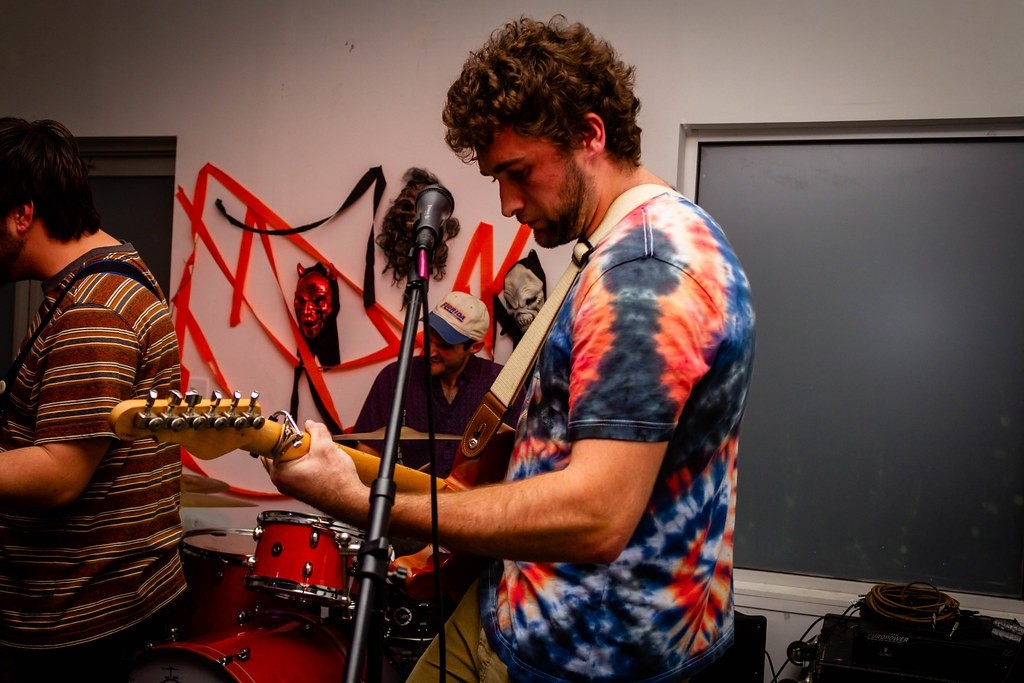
[331,427,461,443]
[181,473,229,496]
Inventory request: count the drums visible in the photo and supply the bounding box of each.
[251,504,392,606]
[125,602,370,683]
[181,529,257,625]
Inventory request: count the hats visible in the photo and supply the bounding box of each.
[418,291,490,345]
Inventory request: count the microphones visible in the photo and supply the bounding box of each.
[411,184,454,254]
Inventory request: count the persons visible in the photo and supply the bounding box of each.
[350,292,526,480]
[0,114,188,683]
[260,18,757,683]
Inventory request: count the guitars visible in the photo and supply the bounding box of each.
[110,384,495,606]
